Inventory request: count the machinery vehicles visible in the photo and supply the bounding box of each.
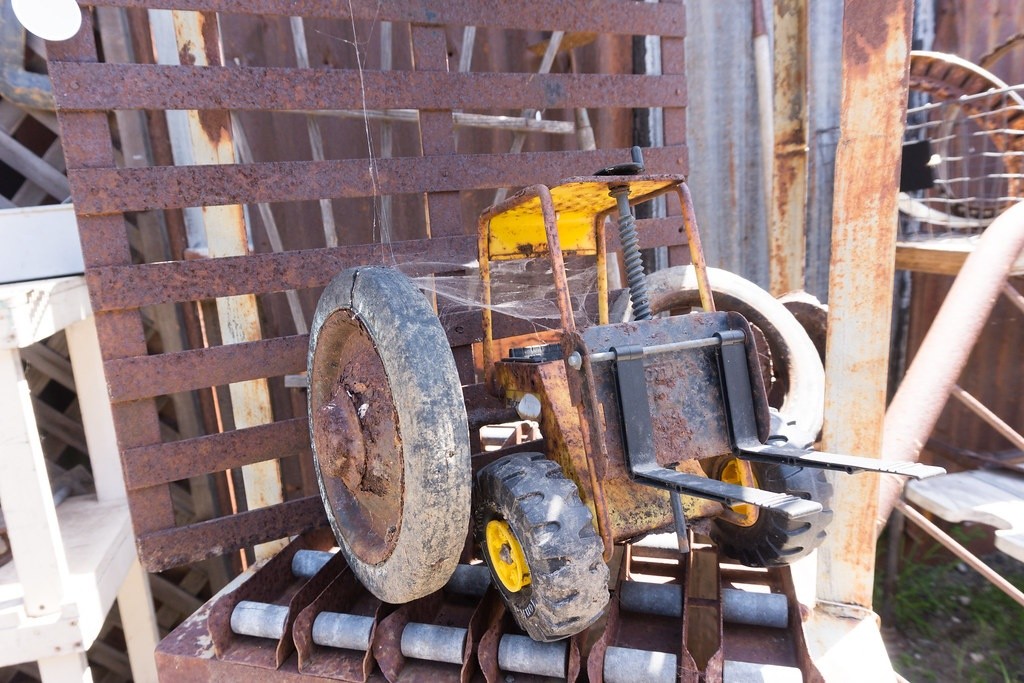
[304,144,948,644]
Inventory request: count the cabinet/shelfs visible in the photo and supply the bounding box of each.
[0,273,160,683]
